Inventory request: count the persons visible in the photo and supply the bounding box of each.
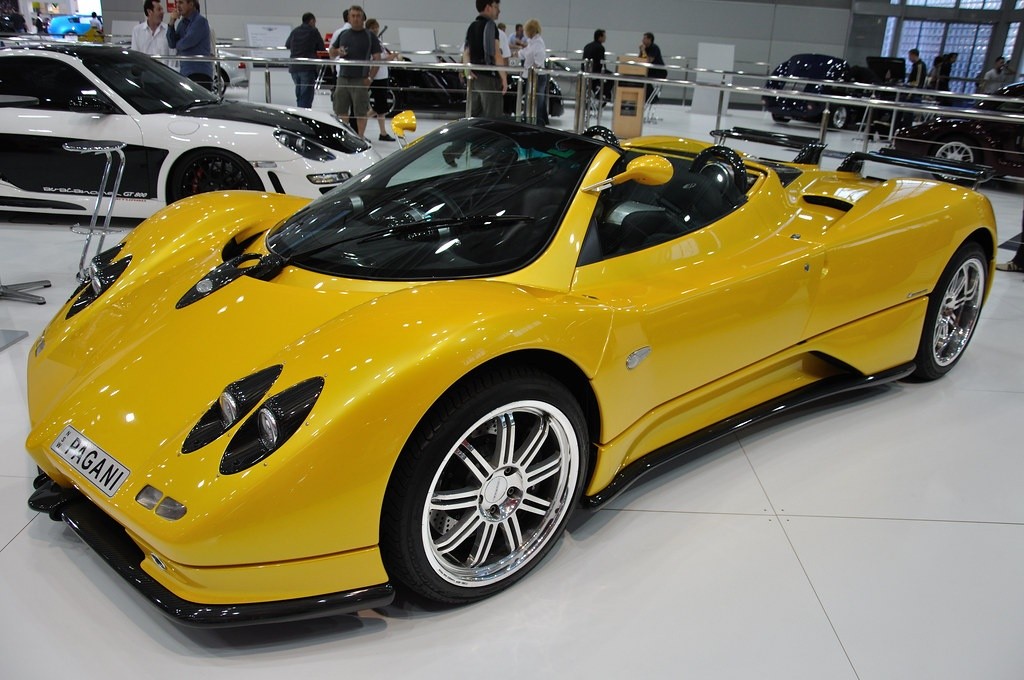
[131,0,174,66]
[35,11,44,33]
[508,24,529,53]
[995,211,1024,271]
[981,57,1015,94]
[498,23,506,32]
[927,52,958,90]
[286,13,326,108]
[329,5,382,137]
[10,7,26,33]
[639,33,667,104]
[581,30,614,100]
[518,19,547,116]
[90,12,102,30]
[365,19,396,141]
[903,49,926,123]
[166,0,214,91]
[442,0,511,169]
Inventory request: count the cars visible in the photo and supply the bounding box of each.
[879,81,1024,188]
[759,51,874,131]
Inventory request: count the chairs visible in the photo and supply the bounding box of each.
[619,171,728,245]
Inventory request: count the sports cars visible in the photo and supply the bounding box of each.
[0,12,26,38]
[46,13,103,41]
[20,113,1002,629]
[0,38,384,224]
[104,41,254,98]
[364,51,566,120]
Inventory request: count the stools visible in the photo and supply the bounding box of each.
[63,140,128,283]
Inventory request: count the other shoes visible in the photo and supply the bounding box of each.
[379,134,395,141]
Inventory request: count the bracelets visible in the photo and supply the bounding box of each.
[368,77,373,82]
[1006,66,1008,69]
